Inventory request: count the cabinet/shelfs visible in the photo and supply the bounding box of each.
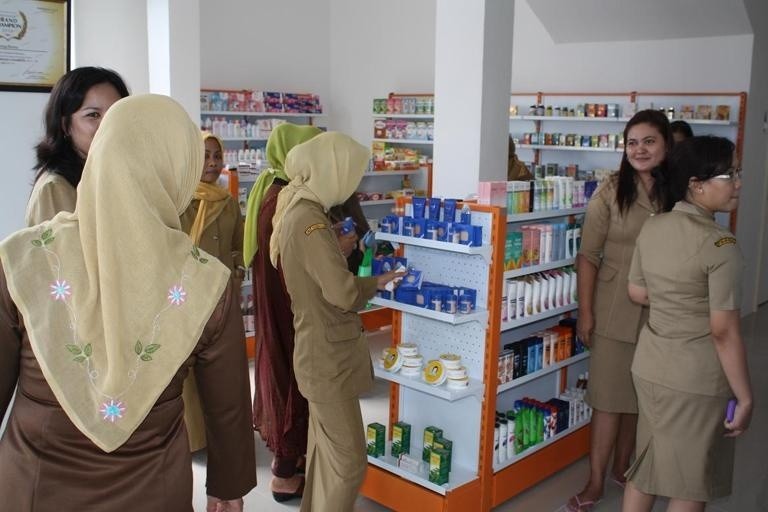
[355,199,506,512]
[201,90,747,356]
[488,198,597,510]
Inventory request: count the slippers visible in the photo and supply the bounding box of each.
[564,495,602,512]
[272,455,306,502]
[609,477,627,488]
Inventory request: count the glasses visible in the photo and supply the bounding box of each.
[711,168,743,181]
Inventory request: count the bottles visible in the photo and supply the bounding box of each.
[536,104,544,116]
[667,107,675,121]
[553,106,561,117]
[529,103,537,116]
[562,106,568,116]
[545,105,552,117]
[239,295,248,337]
[659,107,665,116]
[246,294,255,333]
[569,109,575,116]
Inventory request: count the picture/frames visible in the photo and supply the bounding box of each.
[0,0,71,93]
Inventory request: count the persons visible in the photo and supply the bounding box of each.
[276,132,408,512]
[508,135,536,181]
[568,109,673,512]
[0,93,257,511]
[180,129,243,453]
[243,122,359,502]
[26,67,129,226]
[621,136,755,512]
[668,122,694,142]
[328,194,376,275]
